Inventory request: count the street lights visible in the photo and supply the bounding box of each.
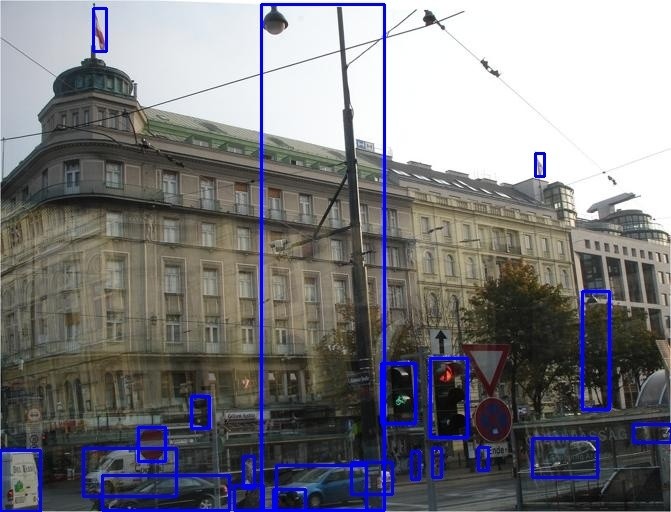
[261,4,384,511]
[582,291,609,409]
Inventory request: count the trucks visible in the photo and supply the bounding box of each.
[84,448,177,496]
[0,450,40,511]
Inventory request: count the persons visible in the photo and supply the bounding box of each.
[273,489,306,511]
[433,449,442,478]
[234,485,264,511]
[243,456,255,488]
[412,451,420,480]
[478,448,489,471]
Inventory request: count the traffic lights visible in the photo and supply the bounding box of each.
[192,396,210,429]
[382,363,416,424]
[430,358,467,438]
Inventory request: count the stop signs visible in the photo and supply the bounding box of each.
[138,427,165,462]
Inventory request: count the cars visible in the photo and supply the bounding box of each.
[277,465,366,510]
[352,463,393,495]
[634,425,671,442]
[102,475,229,511]
[533,439,597,477]
